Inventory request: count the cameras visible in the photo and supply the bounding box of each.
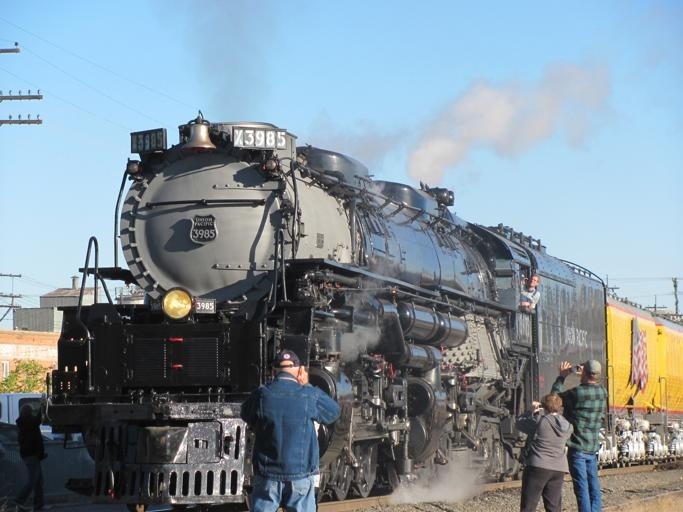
[538,405,543,409]
[570,366,581,373]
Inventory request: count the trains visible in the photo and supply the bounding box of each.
[50,109,683,506]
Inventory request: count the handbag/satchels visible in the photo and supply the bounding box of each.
[518,415,545,464]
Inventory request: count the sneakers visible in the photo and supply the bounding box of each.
[33,504,57,511]
[15,499,32,511]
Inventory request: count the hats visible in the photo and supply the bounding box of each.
[580,359,601,374]
[274,349,300,369]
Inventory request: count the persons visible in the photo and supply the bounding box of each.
[240,347,342,511]
[13,403,52,511]
[518,274,540,309]
[514,392,574,512]
[550,359,607,511]
